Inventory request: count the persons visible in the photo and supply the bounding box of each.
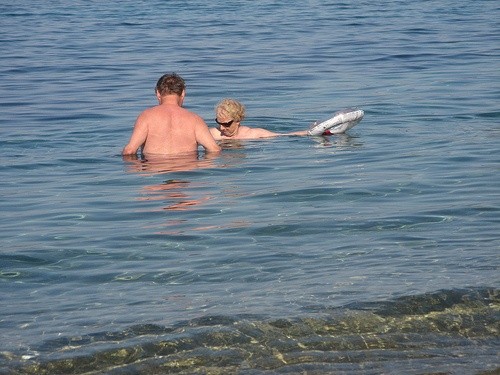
[121,73,221,155]
[209,98,309,141]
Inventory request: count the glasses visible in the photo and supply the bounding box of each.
[215,118,233,127]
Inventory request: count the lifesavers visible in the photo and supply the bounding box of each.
[308,109,364,136]
